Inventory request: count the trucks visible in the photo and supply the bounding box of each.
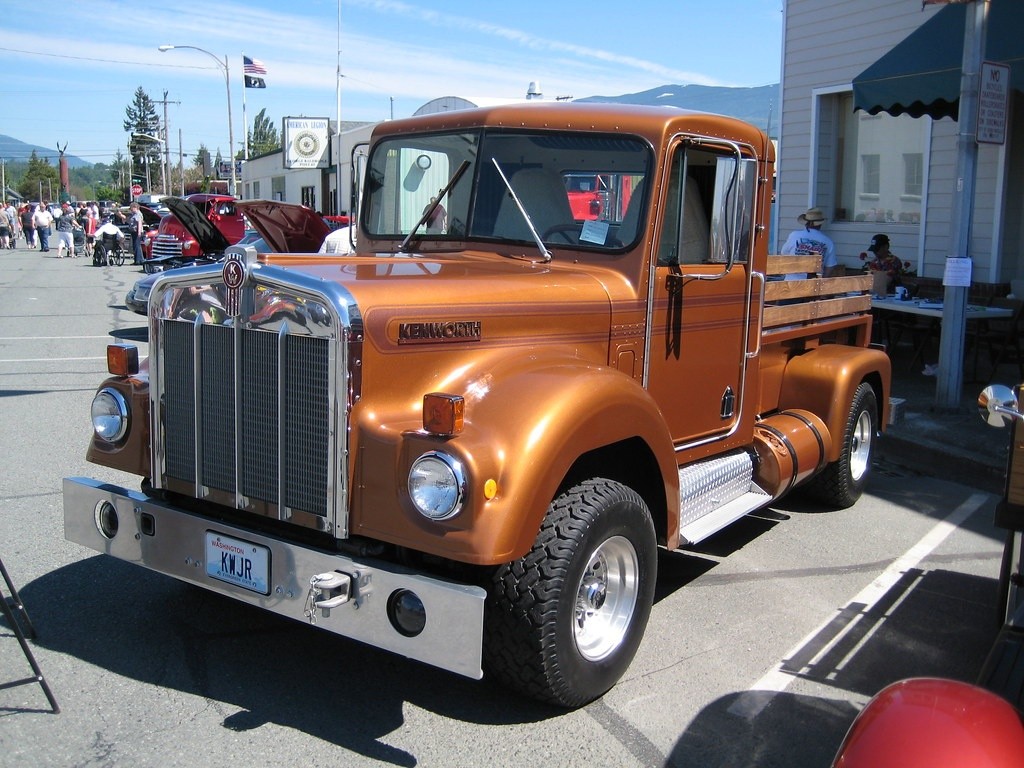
[63,101,892,710]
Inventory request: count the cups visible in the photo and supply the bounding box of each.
[895,286,908,300]
[975,296,984,310]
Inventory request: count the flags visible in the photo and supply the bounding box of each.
[244,55,268,88]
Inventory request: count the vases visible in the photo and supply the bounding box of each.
[867,271,887,300]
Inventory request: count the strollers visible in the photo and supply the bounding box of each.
[9,225,16,248]
[67,225,89,257]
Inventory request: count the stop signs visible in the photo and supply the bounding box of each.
[131,184,143,196]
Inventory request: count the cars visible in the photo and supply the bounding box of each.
[29,200,117,219]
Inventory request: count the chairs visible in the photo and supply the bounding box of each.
[614,172,709,265]
[491,167,580,246]
[879,278,1024,371]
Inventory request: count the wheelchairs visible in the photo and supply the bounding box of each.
[93,232,125,267]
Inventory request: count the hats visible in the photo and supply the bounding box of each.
[798,206,826,233]
[866,233,890,252]
[66,208,74,215]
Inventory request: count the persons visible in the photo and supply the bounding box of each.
[860,234,902,294]
[316,211,330,229]
[0,199,146,267]
[423,197,447,234]
[782,205,837,282]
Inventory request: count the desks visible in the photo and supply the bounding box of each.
[835,293,1013,383]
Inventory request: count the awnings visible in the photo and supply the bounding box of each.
[850,0,1024,129]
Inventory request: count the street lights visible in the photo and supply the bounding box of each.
[157,45,236,195]
[105,168,125,200]
[98,181,116,191]
[132,133,172,197]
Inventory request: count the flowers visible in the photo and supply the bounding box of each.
[859,252,911,271]
